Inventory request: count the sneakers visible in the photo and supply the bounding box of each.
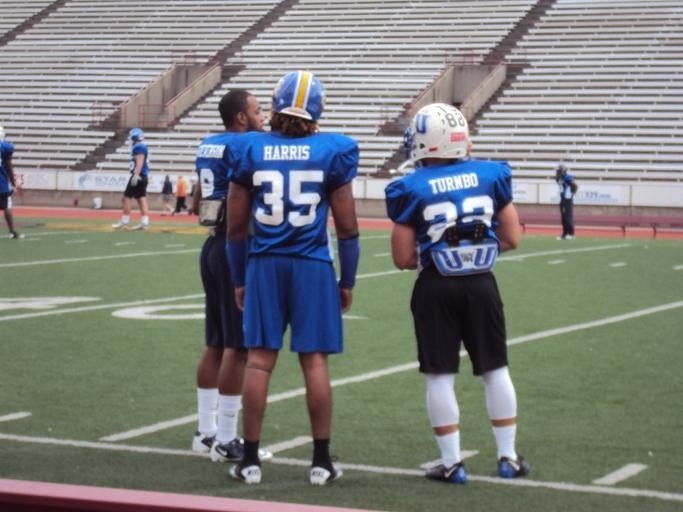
[192,431,273,484]
[113,222,127,228]
[426,463,466,484]
[496,455,530,476]
[309,454,342,486]
[134,223,147,230]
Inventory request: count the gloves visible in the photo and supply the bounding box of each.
[131,175,141,186]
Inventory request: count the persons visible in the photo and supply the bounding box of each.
[111,127,150,230]
[221,70,360,488]
[0,127,25,240]
[384,101,530,485]
[191,90,273,466]
[172,175,188,215]
[554,164,579,240]
[159,174,173,217]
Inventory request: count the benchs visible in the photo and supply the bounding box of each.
[1,3,683,185]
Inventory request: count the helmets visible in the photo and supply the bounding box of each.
[404,103,470,161]
[130,128,143,140]
[272,71,325,122]
